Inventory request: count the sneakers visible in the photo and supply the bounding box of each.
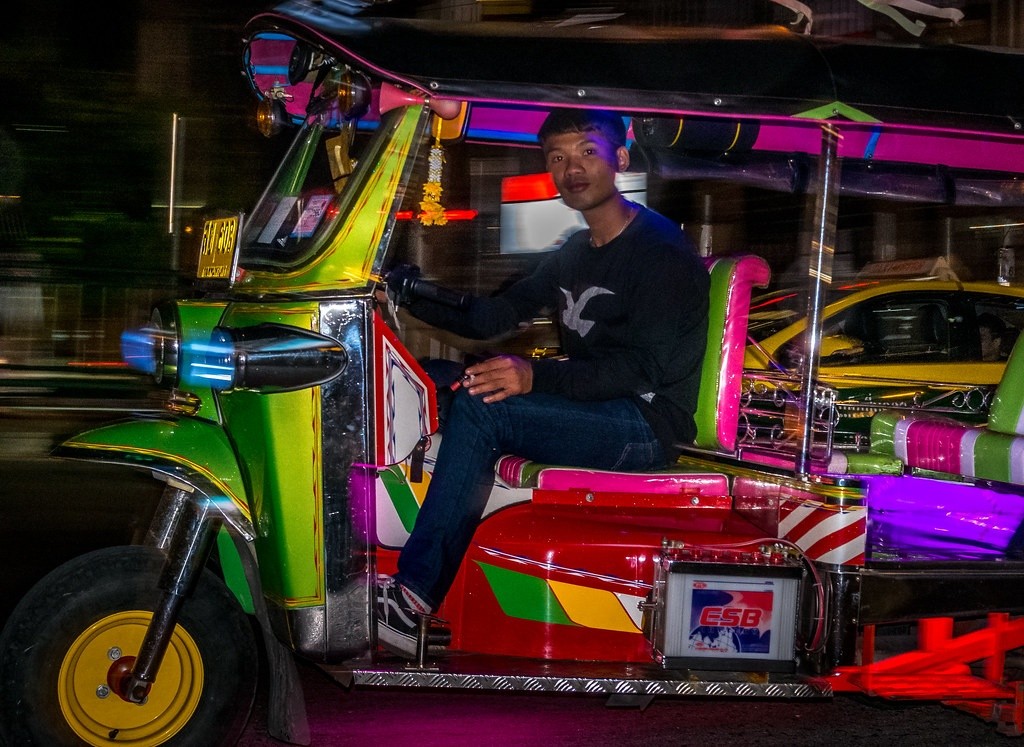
[377,577,420,659]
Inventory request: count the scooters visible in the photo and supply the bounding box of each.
[1,1,1024,747]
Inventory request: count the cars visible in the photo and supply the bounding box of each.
[743,276,1024,416]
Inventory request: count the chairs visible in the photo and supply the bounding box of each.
[495,252,770,501]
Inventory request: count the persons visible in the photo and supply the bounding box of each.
[356,97,713,650]
[978,312,1011,363]
